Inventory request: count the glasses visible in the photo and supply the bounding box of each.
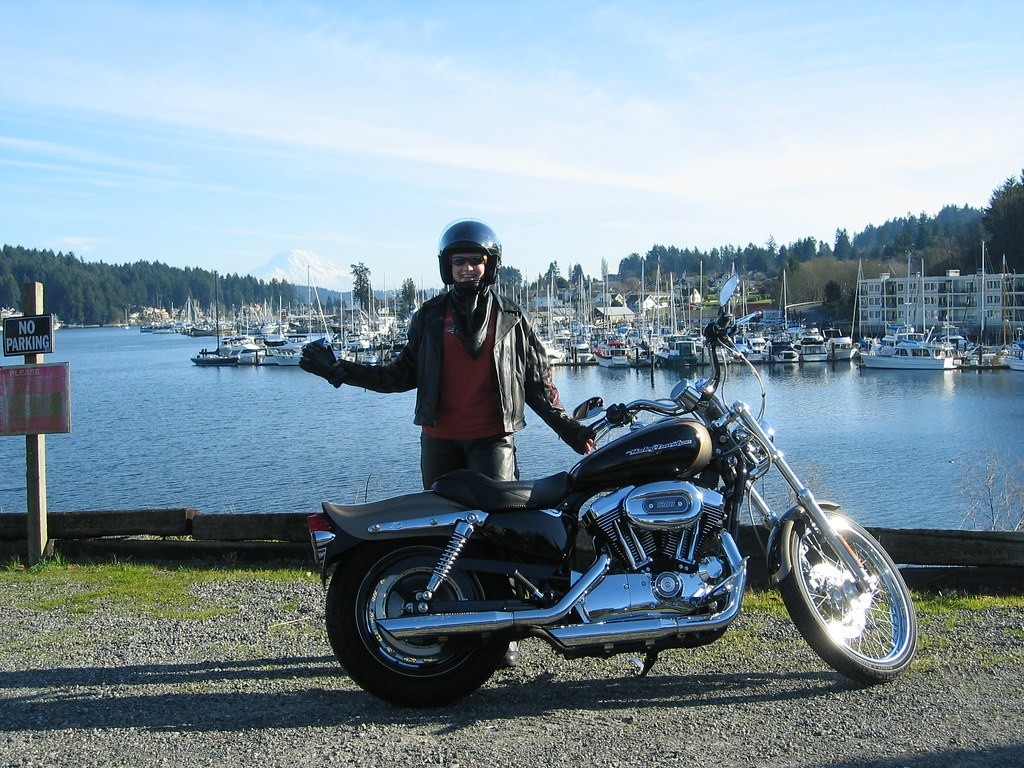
[449,255,486,267]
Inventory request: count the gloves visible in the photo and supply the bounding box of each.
[299,341,348,389]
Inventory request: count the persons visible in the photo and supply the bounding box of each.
[333,220,598,667]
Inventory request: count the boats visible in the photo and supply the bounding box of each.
[50,313,64,331]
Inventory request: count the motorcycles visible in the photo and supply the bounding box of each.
[305,277,918,711]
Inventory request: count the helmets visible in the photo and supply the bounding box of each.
[438,221,501,285]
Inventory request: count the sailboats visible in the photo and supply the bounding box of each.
[138,240,1023,371]
[123,309,130,330]
